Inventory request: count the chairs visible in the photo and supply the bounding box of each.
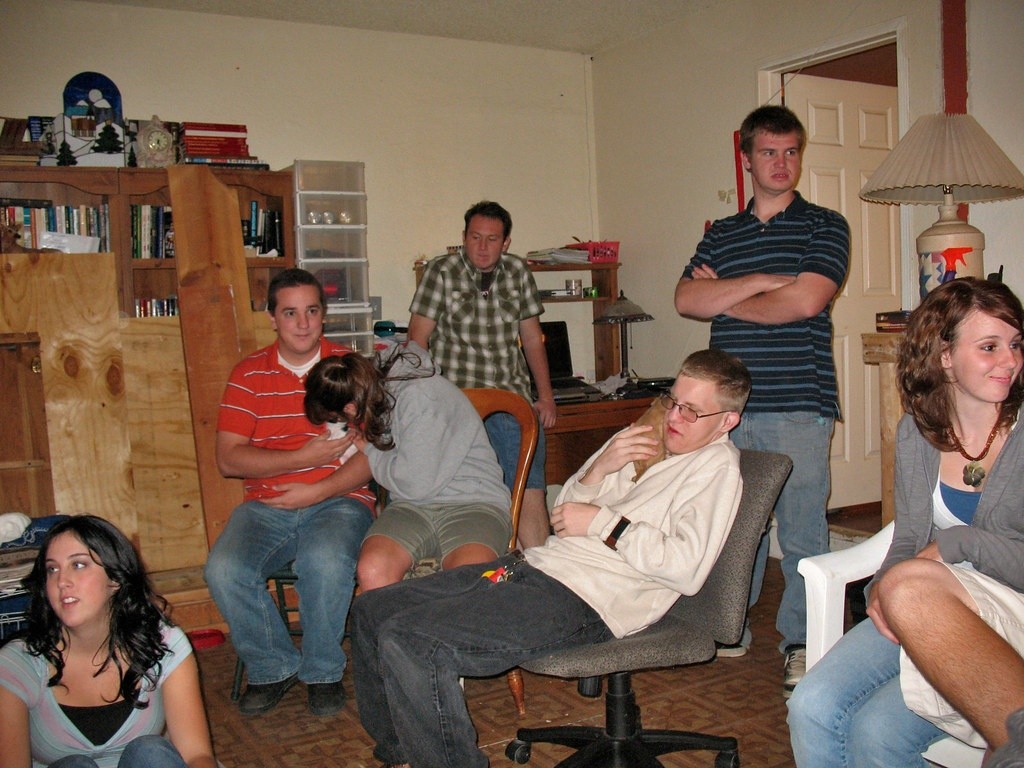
[798,520,984,768]
[505,450,794,768]
[227,550,357,700]
[381,384,536,716]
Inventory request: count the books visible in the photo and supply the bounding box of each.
[0,119,41,165]
[178,122,270,170]
[130,204,174,258]
[135,297,178,318]
[527,248,589,264]
[242,201,282,256]
[0,204,111,253]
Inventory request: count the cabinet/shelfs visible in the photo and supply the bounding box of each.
[294,159,372,362]
[0,168,291,516]
[413,259,625,387]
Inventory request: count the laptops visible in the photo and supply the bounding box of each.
[521,321,602,403]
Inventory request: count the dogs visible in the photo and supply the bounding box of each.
[325,420,359,464]
[632,393,671,483]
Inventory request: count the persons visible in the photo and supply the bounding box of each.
[787,276,1024,768]
[877,557,1024,768]
[407,201,557,550]
[304,341,514,591]
[674,107,850,698]
[352,349,753,768]
[0,516,218,768]
[202,268,376,716]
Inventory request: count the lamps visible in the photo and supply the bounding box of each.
[592,291,651,382]
[860,111,1024,303]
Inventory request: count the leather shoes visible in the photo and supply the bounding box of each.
[238,672,298,717]
[307,681,348,717]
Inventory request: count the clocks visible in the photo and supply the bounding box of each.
[131,112,178,166]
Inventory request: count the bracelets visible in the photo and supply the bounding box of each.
[604,516,631,551]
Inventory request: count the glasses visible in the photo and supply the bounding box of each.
[659,392,736,423]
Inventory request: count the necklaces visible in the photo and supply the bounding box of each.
[948,415,1003,488]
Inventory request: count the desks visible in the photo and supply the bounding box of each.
[525,392,667,482]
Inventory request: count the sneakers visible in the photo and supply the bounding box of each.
[782,648,806,698]
[713,641,747,657]
